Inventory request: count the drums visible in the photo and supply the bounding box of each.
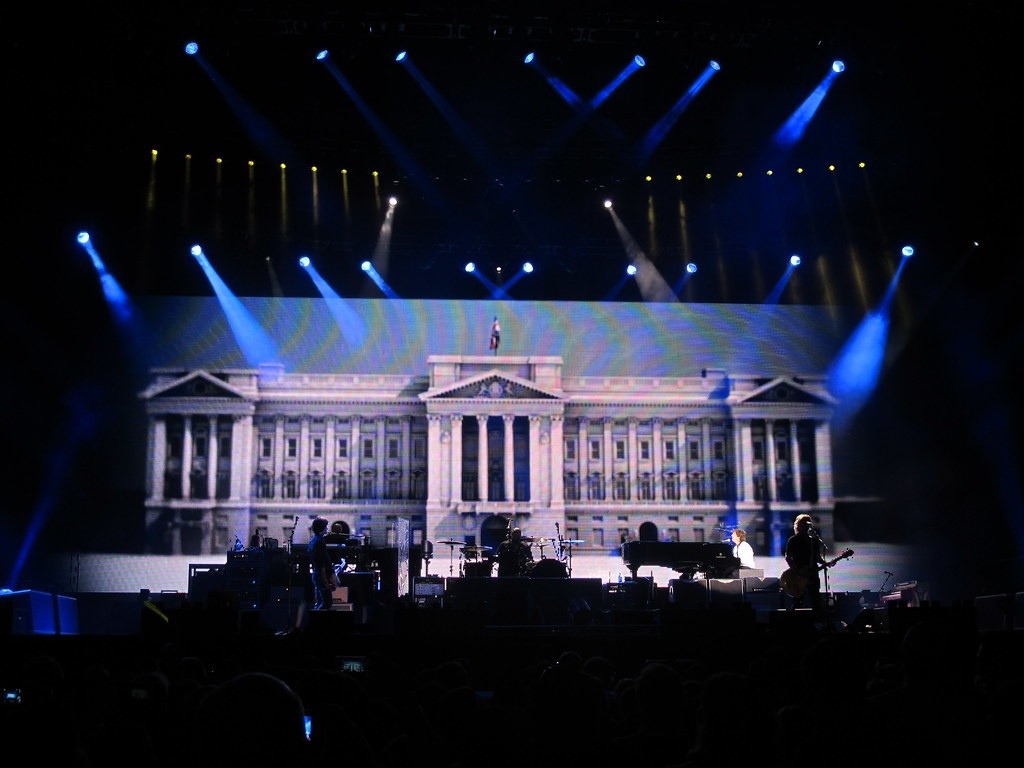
[464,562,492,577]
[530,558,568,578]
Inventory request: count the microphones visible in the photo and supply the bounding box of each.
[555,521,559,526]
[884,571,893,576]
[296,515,300,519]
[806,521,812,525]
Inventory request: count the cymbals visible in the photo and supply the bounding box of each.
[459,546,493,551]
[559,539,585,543]
[437,541,467,544]
[521,536,554,547]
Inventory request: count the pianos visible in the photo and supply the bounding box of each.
[621,540,736,580]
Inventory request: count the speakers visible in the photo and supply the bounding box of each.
[670,577,782,612]
[850,606,887,633]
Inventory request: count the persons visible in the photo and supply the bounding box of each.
[731,530,754,579]
[1,623,1024,768]
[781,514,827,590]
[309,519,349,609]
[233,539,245,558]
[496,527,534,577]
[251,529,261,547]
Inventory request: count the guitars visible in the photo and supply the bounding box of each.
[779,548,855,598]
